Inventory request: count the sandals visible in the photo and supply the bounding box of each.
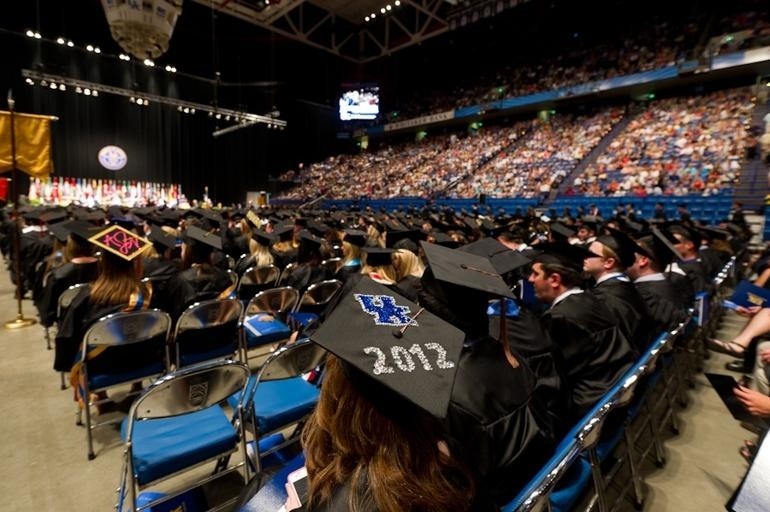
[704,336,745,358]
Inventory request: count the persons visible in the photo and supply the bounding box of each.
[1,203,755,511]
[708,60,770,510]
[258,22,756,198]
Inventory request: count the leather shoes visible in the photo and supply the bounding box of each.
[726,361,749,371]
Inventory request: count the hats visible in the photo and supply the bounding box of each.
[88,226,154,261]
[550,222,575,239]
[274,209,327,233]
[180,207,274,226]
[72,206,180,225]
[185,226,222,255]
[662,221,701,249]
[299,232,322,252]
[644,226,685,266]
[311,275,466,434]
[419,240,518,368]
[464,215,498,238]
[362,247,403,266]
[344,227,369,247]
[597,226,650,267]
[532,239,601,272]
[384,213,464,248]
[581,214,643,232]
[148,226,178,252]
[24,204,72,242]
[252,230,281,248]
[61,219,102,240]
[458,238,533,275]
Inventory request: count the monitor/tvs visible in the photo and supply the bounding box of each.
[335,81,383,123]
